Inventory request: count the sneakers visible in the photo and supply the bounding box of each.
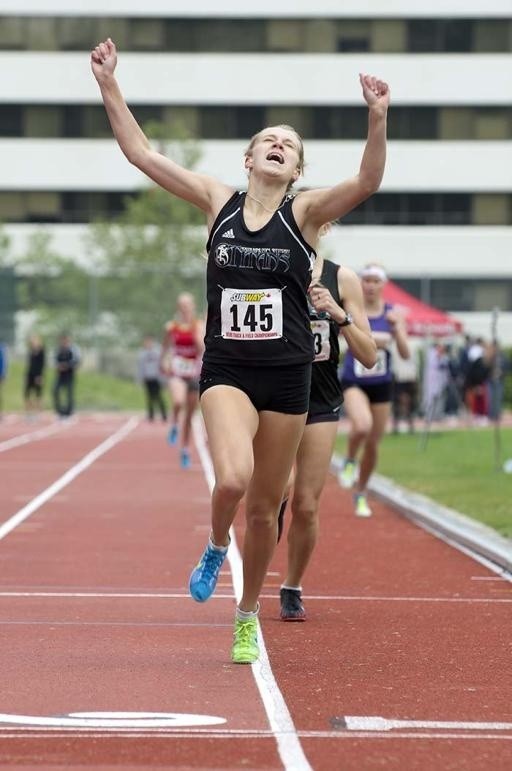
[279,588,306,621]
[168,426,178,445]
[181,451,189,469]
[340,457,355,491]
[230,615,260,663]
[351,495,373,517]
[189,542,228,602]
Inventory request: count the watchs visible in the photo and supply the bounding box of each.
[336,311,354,327]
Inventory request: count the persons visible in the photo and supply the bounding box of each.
[334,262,411,522]
[273,186,380,623]
[390,328,504,439]
[136,330,169,424]
[159,292,202,469]
[50,330,84,418]
[88,37,393,668]
[21,325,50,422]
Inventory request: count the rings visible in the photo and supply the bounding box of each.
[316,292,322,301]
